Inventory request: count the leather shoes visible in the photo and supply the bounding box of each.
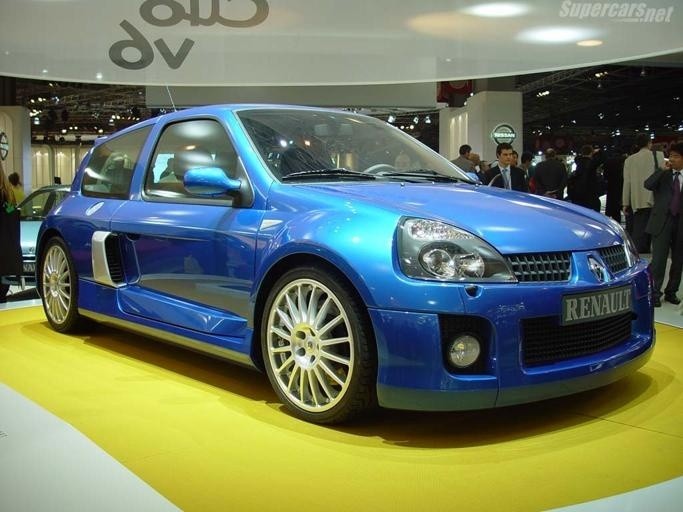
[664,295,680,305]
[654,298,661,306]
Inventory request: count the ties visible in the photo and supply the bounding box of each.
[670,172,681,216]
[501,168,509,190]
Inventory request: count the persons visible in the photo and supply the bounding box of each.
[0,153,31,303]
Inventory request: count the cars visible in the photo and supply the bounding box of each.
[25,83,660,428]
[0,184,72,302]
[562,161,627,228]
